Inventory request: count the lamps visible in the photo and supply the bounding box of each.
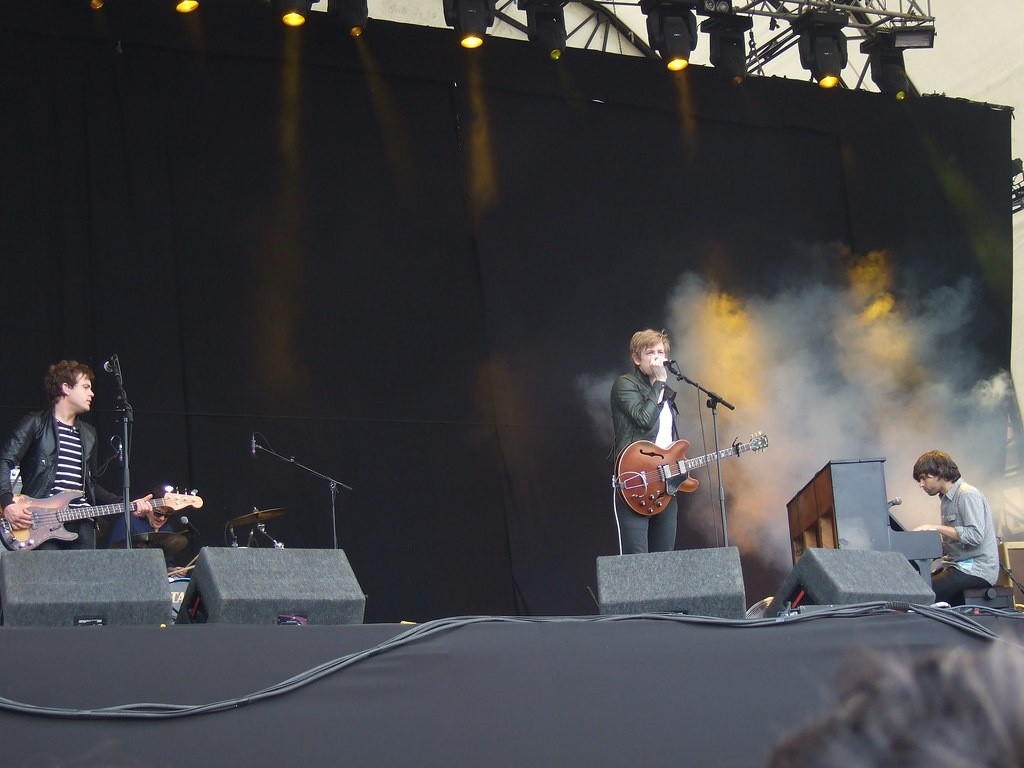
[271,0,911,107]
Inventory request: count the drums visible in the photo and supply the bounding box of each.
[167,579,189,624]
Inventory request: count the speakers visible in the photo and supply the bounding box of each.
[596,547,747,619]
[996,541,1024,609]
[0,547,173,627]
[761,548,937,620]
[174,546,365,626]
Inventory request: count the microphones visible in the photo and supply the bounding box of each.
[119,439,123,462]
[104,354,118,372]
[180,516,200,535]
[230,523,234,537]
[663,360,676,366]
[252,434,256,453]
[887,497,903,508]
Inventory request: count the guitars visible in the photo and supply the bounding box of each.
[1,485,204,551]
[614,432,769,518]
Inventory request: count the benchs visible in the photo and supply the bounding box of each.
[953,585,1015,610]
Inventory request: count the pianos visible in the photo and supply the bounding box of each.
[787,457,942,594]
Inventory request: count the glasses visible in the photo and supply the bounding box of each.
[154,509,172,519]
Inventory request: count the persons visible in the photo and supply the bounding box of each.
[912,449,999,608]
[0,360,153,550]
[606,329,681,555]
[107,486,187,577]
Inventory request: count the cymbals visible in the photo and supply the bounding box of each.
[228,505,288,526]
[95,515,111,540]
[109,530,189,555]
